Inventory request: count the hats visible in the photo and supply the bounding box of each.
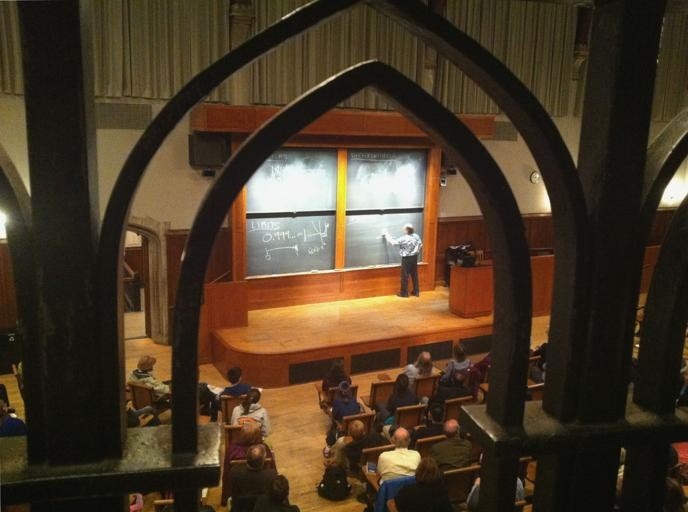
[137,355,156,370]
[339,381,350,391]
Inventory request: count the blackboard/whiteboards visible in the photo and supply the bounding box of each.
[242,144,434,280]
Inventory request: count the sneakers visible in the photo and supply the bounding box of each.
[323,448,331,458]
[357,493,371,504]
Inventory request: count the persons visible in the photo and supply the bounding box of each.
[316,303,688,511]
[0,355,303,512]
[383,224,424,299]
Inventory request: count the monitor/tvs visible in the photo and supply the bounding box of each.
[462,256,476,267]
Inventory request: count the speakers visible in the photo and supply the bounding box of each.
[442,151,454,168]
[188,133,231,169]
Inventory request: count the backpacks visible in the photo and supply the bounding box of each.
[318,462,351,500]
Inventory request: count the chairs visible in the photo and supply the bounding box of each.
[314,352,547,511]
[126,380,273,512]
